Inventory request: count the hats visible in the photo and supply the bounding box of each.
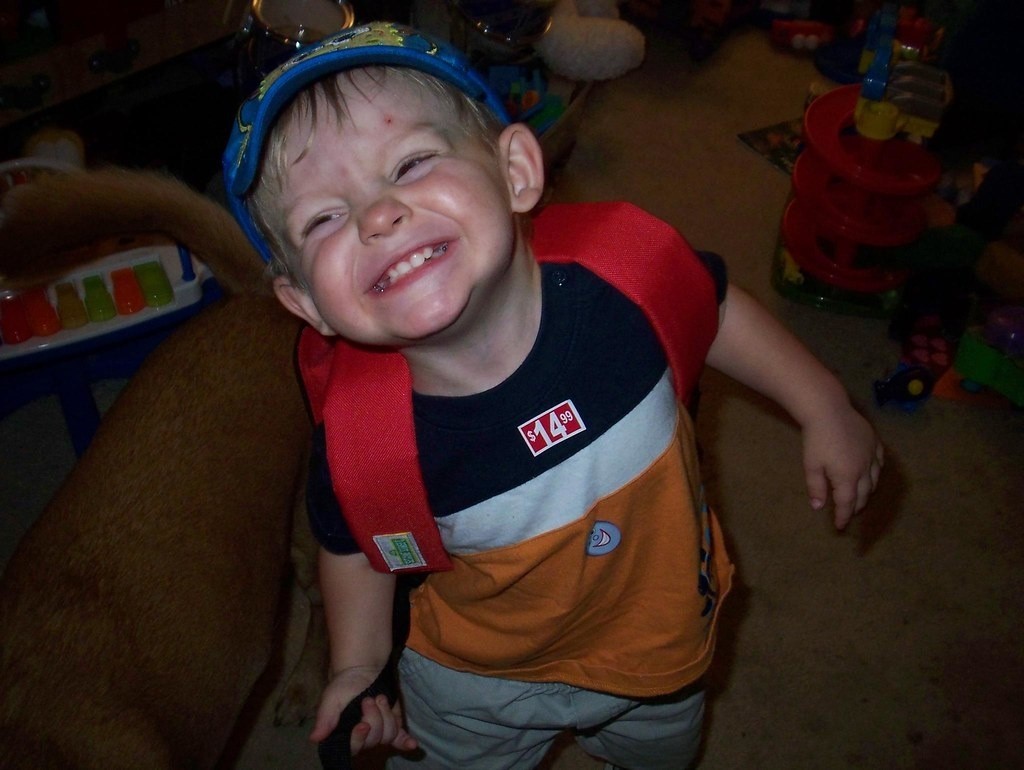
[224,22,512,271]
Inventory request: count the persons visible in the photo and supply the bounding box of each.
[222,20,886,770]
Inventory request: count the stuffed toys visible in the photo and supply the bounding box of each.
[2,168,319,770]
[530,0,647,82]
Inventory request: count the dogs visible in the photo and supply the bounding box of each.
[1,162,313,770]
